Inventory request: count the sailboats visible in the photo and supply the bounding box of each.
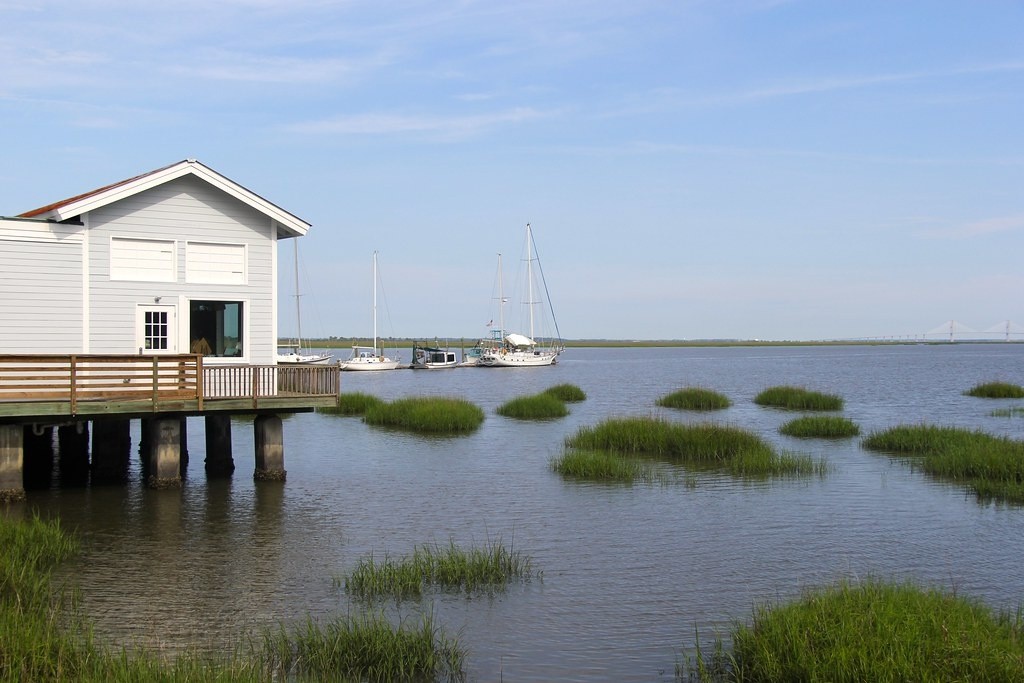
[465,252,530,366]
[478,223,566,366]
[278,236,335,364]
[337,250,401,371]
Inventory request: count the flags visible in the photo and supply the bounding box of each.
[487,321,493,327]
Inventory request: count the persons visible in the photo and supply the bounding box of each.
[190,332,212,354]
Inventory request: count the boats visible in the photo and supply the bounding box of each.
[409,338,460,368]
